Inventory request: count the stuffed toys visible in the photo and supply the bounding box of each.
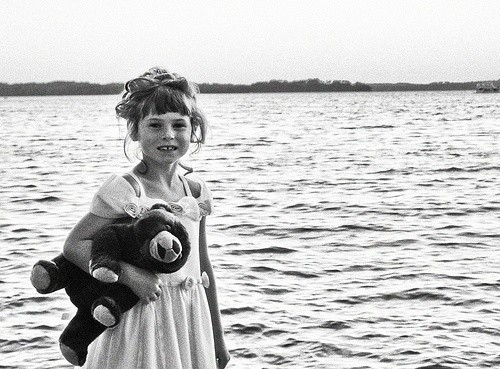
[30,203,190,365]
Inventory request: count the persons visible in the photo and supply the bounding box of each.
[62,65,231,369]
